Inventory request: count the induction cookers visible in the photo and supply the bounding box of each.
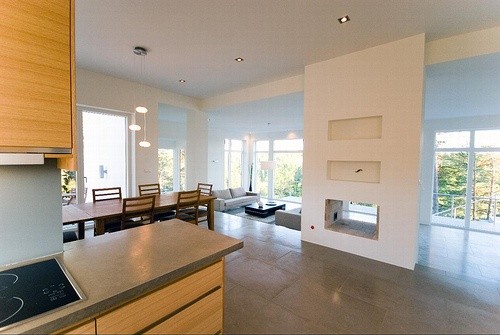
[0,256,88,332]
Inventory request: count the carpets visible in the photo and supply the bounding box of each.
[225,205,275,225]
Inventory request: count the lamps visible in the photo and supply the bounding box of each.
[99,164,107,178]
[129,46,151,148]
[260,159,276,170]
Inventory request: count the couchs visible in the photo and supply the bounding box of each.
[275,203,301,231]
[212,187,260,212]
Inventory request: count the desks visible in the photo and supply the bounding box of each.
[62,204,93,240]
[74,191,218,235]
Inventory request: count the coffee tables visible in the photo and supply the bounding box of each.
[245,201,286,218]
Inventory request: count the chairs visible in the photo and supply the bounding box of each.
[120,195,156,230]
[166,190,202,225]
[138,183,161,220]
[92,187,124,203]
[197,183,213,223]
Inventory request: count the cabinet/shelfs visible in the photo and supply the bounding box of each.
[0,0,78,174]
[50,254,224,335]
